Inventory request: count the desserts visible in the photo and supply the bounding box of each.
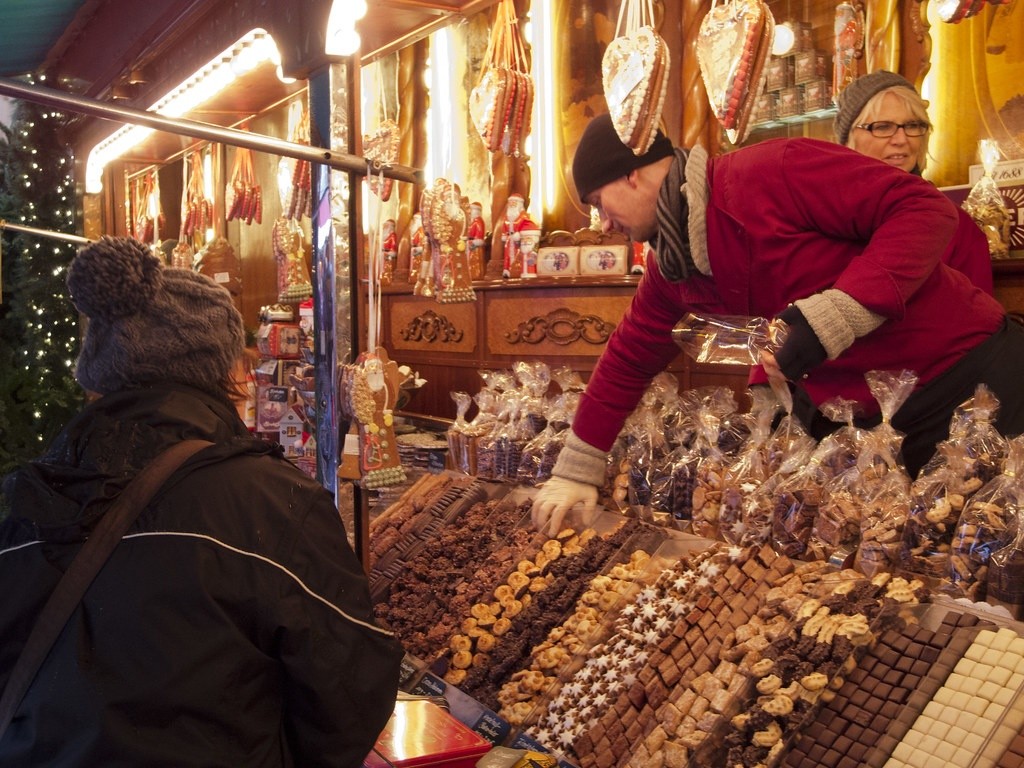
[366,410,1024,768]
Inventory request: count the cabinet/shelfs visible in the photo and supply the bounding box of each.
[23,0,1024,768]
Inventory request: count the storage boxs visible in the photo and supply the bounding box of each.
[244,323,317,482]
[538,246,630,276]
[753,23,836,129]
[362,691,493,768]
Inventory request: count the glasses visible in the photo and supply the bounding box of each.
[856,120,929,137]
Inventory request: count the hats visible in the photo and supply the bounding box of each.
[67,236,244,389]
[832,70,916,144]
[573,114,675,205]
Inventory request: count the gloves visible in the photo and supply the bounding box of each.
[531,476,598,538]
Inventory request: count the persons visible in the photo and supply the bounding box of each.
[833,68,933,176]
[382,194,541,283]
[0,232,401,768]
[527,111,1024,539]
